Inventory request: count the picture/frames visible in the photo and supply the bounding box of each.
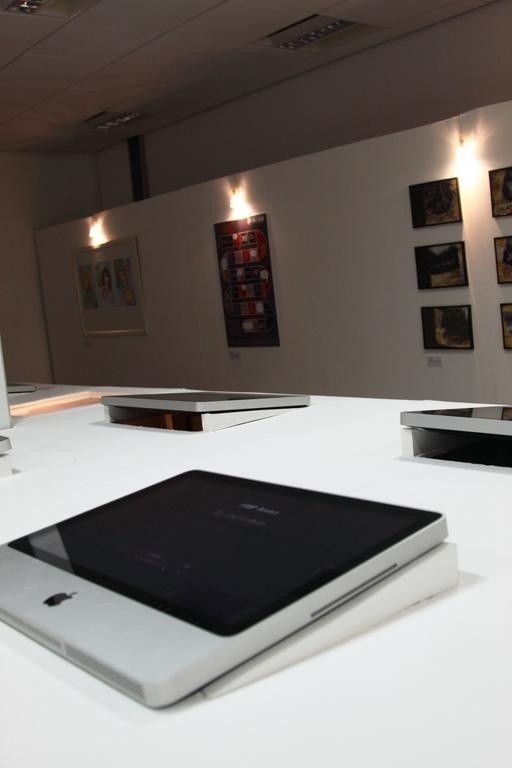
[499,302,512,350]
[414,240,469,291]
[70,234,149,338]
[420,304,474,350]
[407,175,464,230]
[485,166,512,217]
[493,236,512,284]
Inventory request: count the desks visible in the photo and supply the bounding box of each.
[0,382,512,768]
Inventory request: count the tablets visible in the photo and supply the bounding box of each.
[6,382,36,393]
[400,405,512,439]
[0,435,11,456]
[1,469,448,708]
[102,392,310,412]
